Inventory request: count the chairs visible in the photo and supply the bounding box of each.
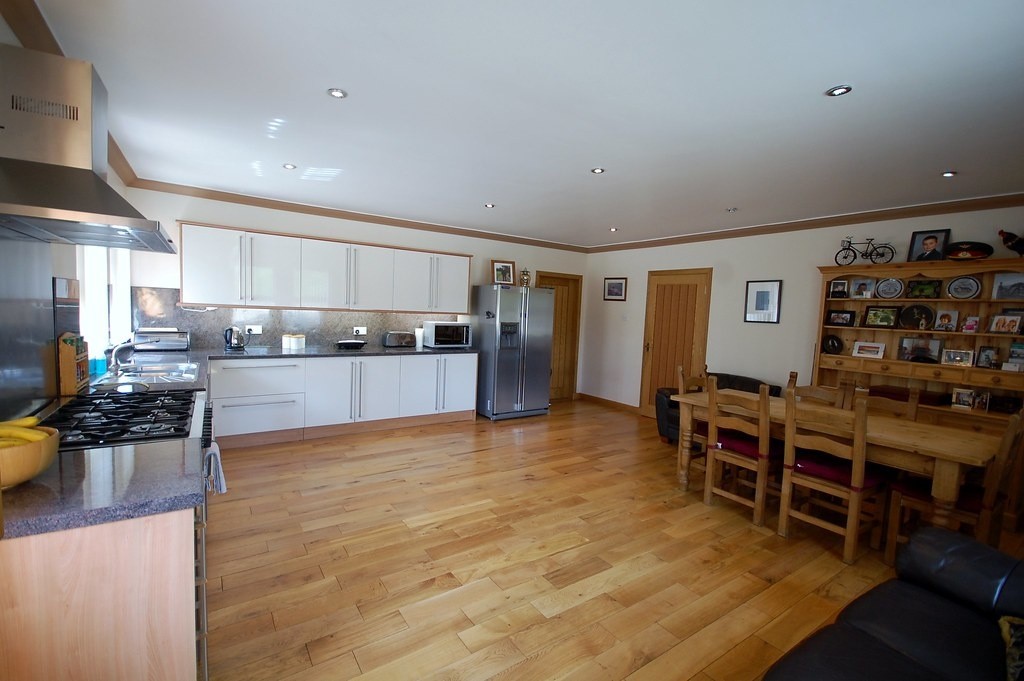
[678,363,1023,569]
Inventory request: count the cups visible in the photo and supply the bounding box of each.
[281,334,305,350]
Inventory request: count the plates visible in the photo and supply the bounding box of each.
[874,278,904,299]
[946,276,981,299]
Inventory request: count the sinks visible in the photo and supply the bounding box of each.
[95,362,200,384]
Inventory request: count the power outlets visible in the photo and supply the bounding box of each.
[353,327,367,335]
[244,324,262,335]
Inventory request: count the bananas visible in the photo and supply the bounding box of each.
[0,416,50,447]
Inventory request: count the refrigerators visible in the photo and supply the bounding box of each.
[471,285,555,420]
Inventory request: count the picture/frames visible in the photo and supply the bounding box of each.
[896,336,945,363]
[975,346,1000,368]
[991,272,1024,299]
[490,259,516,285]
[984,313,1024,334]
[603,277,627,301]
[906,229,951,262]
[941,349,973,366]
[831,279,847,297]
[904,279,942,297]
[825,310,854,326]
[951,387,975,411]
[852,341,885,359]
[861,305,902,329]
[849,278,876,298]
[744,280,783,324]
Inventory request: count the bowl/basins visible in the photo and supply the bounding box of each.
[0,426,60,491]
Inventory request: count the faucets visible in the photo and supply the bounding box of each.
[109,338,161,375]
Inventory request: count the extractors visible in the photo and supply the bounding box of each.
[0,42,178,254]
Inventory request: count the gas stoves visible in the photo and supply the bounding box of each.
[36,388,212,451]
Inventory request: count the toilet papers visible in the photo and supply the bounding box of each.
[415,328,424,348]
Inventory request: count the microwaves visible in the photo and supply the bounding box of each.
[423,320,473,349]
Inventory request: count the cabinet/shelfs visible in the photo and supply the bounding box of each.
[178,222,477,437]
[811,256,1024,434]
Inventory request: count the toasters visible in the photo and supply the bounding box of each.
[382,331,416,347]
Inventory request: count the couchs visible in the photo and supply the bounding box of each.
[762,528,1024,681]
[655,371,783,444]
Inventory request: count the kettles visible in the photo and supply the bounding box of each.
[223,326,244,351]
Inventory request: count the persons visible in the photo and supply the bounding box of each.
[502,268,507,281]
[831,314,849,323]
[916,236,941,260]
[994,317,1016,331]
[937,314,954,328]
[854,283,867,295]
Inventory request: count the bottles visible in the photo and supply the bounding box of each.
[998,229,1024,256]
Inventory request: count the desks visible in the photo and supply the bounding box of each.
[670,388,1004,533]
